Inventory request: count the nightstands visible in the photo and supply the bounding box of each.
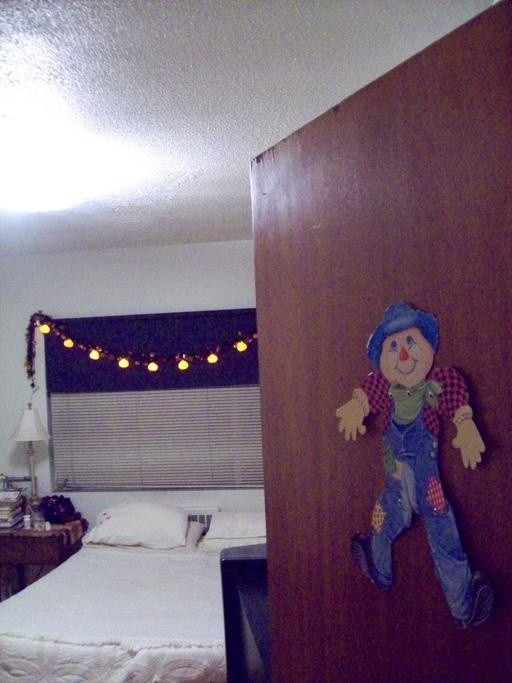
[0,517,88,602]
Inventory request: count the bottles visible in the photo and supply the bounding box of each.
[23,515,31,529]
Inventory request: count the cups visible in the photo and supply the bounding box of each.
[32,511,45,530]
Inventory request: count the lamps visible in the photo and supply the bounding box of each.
[8,407,50,506]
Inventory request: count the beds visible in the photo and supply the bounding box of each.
[1,515,267,683]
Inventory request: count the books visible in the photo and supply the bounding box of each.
[0,486,26,534]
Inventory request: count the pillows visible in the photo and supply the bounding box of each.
[200,511,266,552]
[83,503,206,555]
